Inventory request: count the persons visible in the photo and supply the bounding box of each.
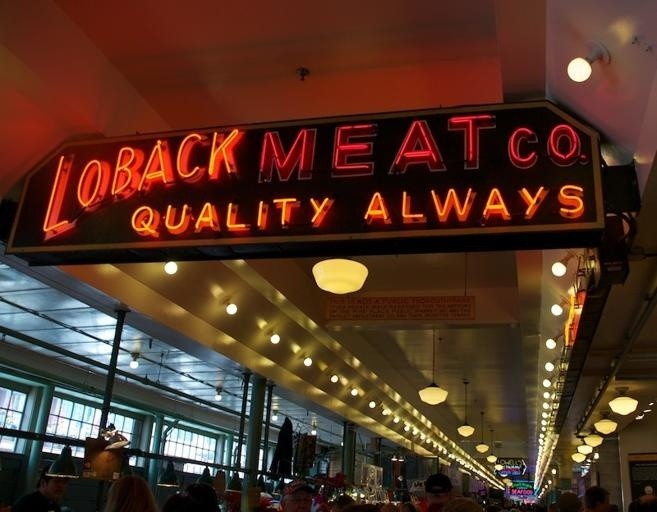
[1,469,657,512]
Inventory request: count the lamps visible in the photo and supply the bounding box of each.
[457,378,500,465]
[391,454,398,462]
[223,299,238,316]
[42,441,287,494]
[265,328,281,345]
[310,256,371,297]
[565,40,612,84]
[417,328,449,407]
[532,249,640,466]
[326,368,339,384]
[129,352,318,435]
[299,352,313,367]
[347,383,358,396]
[397,456,405,462]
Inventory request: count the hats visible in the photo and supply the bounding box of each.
[424,473,454,495]
[282,480,314,496]
